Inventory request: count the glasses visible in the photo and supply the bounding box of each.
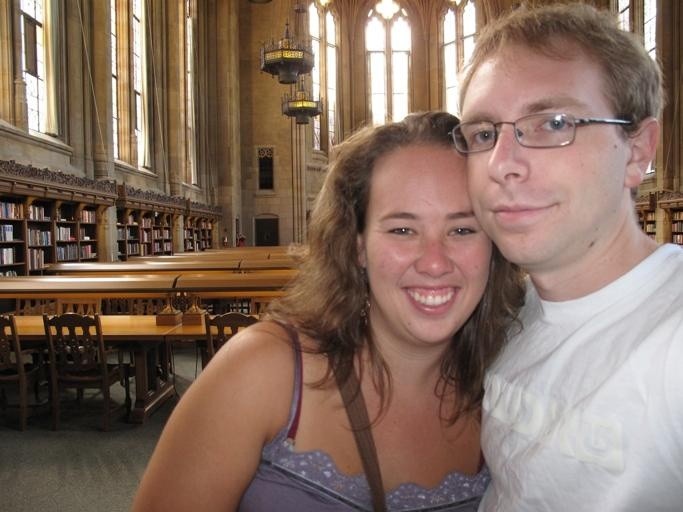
[452,112,631,154]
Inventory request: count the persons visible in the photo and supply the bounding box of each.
[128,110,528,512]
[459,1,683,512]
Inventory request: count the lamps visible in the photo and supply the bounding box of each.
[259,16,314,85]
[281,89,324,125]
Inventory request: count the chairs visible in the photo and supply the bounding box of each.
[1,313,46,429]
[205,312,260,362]
[41,313,135,430]
[56,296,101,314]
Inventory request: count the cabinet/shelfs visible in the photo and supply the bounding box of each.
[635,188,682,245]
[188,197,224,251]
[1,160,118,274]
[119,181,186,257]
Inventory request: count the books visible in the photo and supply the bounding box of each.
[1,203,212,278]
[636,211,683,244]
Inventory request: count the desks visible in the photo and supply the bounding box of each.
[4,314,264,426]
